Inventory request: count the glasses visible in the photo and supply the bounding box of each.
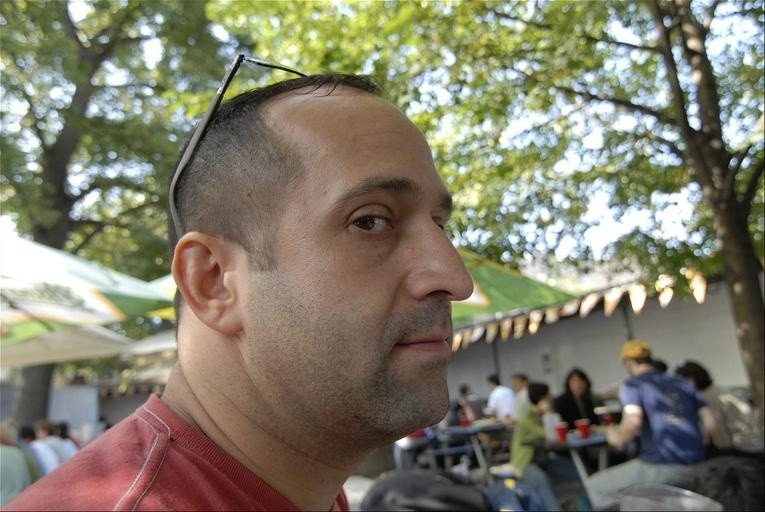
[168,53,308,240]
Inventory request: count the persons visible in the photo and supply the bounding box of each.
[1,410,113,505]
[511,366,604,471]
[402,372,535,468]
[0,73,475,511]
[583,339,741,512]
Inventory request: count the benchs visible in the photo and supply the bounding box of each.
[401,420,740,512]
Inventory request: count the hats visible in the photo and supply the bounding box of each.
[617,339,650,365]
[528,383,549,405]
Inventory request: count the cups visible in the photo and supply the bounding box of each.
[574,420,589,438]
[554,423,568,442]
